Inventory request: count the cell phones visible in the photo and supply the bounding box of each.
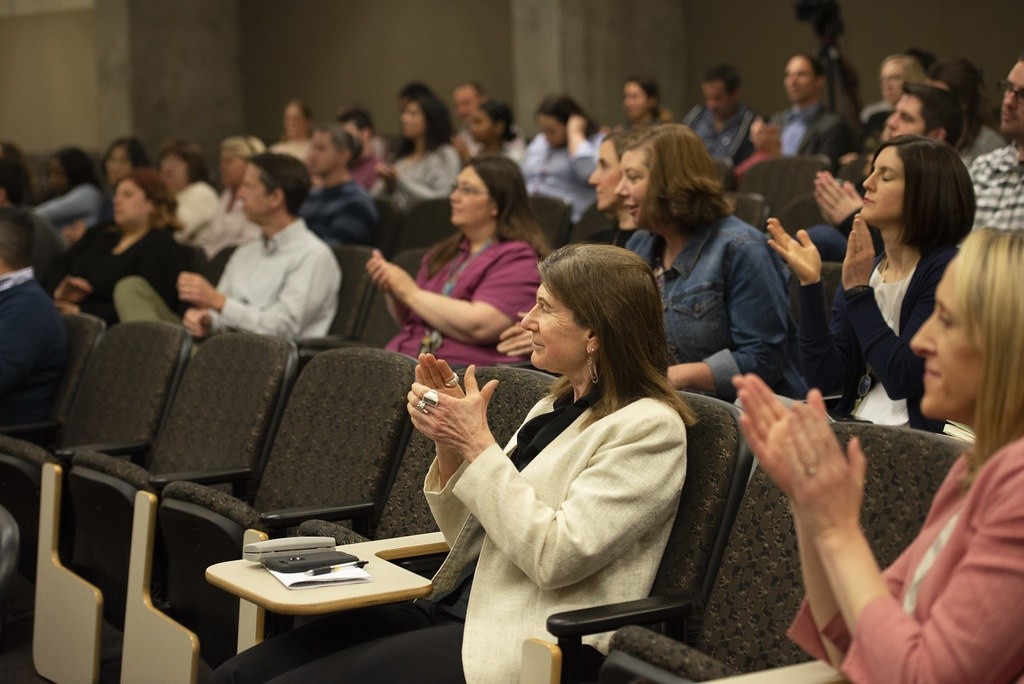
[264,551,360,573]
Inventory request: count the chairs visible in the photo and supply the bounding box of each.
[0,108,1024,684]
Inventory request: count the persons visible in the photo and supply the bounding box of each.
[212,245,700,684]
[177,150,344,341]
[763,134,976,441]
[364,156,544,370]
[730,224,1024,682]
[0,206,67,447]
[0,48,1024,250]
[497,123,788,409]
[38,171,185,334]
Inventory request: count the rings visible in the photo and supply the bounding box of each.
[445,372,460,388]
[422,389,439,407]
[805,460,821,477]
[416,400,429,415]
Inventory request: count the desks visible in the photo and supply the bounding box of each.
[203,531,442,613]
[31,462,102,684]
[119,490,201,684]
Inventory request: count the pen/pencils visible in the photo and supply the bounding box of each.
[304,560,369,575]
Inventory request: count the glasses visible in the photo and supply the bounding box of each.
[448,181,506,195]
[997,80,1024,99]
